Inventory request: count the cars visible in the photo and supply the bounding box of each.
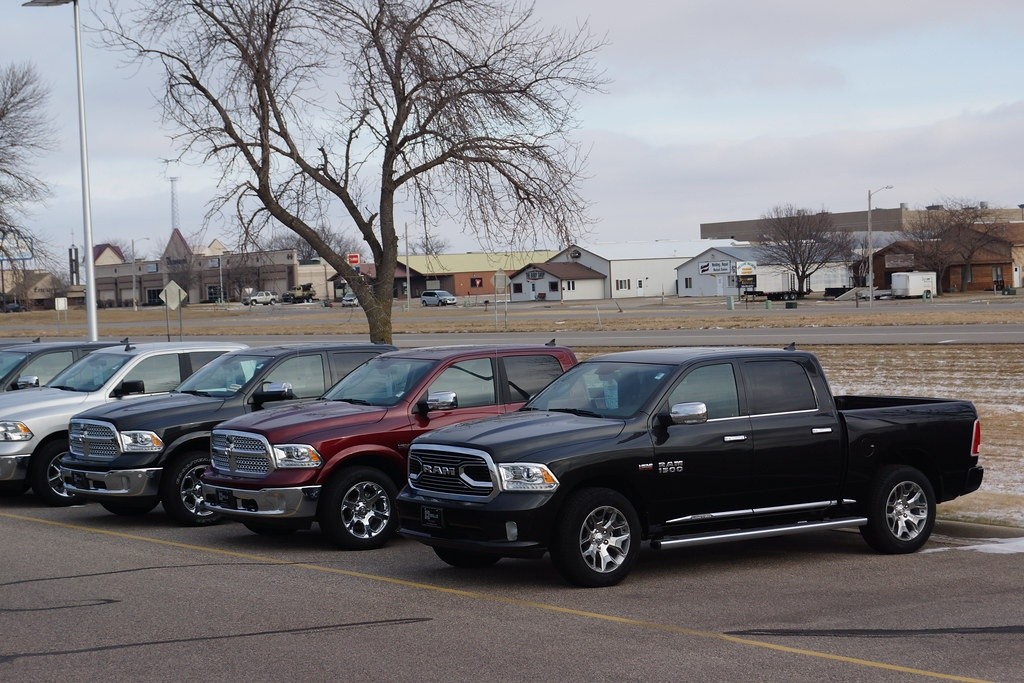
[6,302,30,312]
[855,281,893,302]
[0,339,257,505]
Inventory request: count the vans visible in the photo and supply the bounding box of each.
[420,290,456,306]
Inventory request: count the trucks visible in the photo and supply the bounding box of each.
[281,282,316,303]
[890,270,937,298]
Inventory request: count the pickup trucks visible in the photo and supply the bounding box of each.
[394,340,990,593]
[205,340,591,549]
[243,290,278,307]
[60,333,399,531]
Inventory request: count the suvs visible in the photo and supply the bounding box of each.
[342,290,359,308]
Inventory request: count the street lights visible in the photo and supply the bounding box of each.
[866,184,896,302]
